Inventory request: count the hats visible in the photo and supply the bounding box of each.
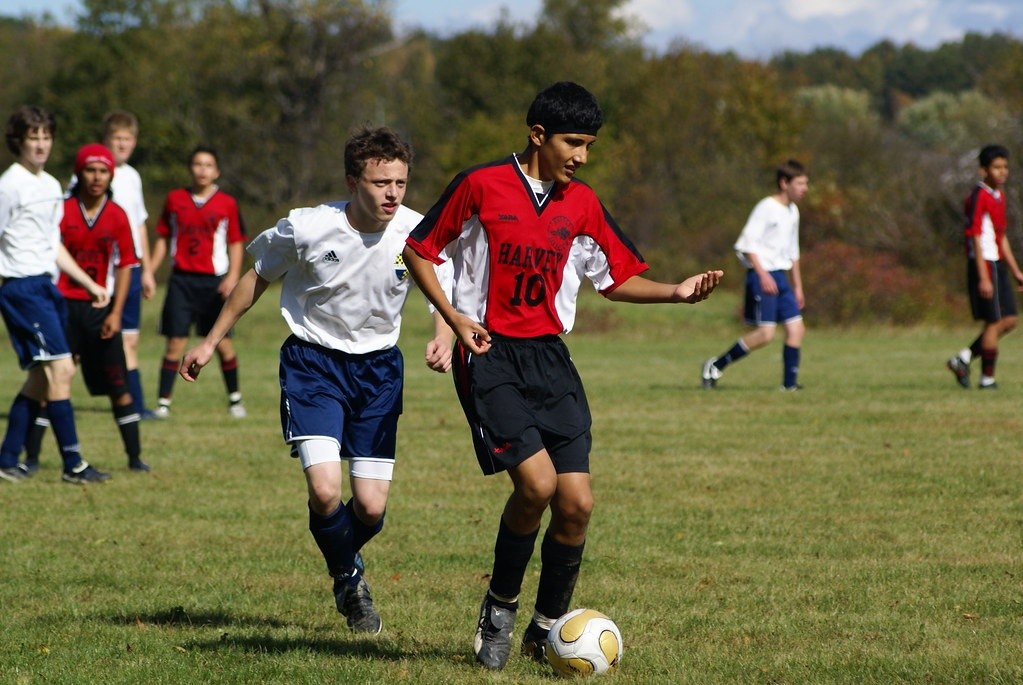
[74,142,114,178]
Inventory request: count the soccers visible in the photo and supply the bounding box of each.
[544,608,623,678]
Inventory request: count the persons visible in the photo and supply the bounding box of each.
[947,145,1023,390]
[152,140,248,420]
[402,81,724,671]
[0,103,111,486]
[15,144,152,472]
[62,113,156,422]
[178,128,454,636]
[704,159,808,393]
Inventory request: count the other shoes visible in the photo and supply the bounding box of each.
[156,400,171,418]
[140,408,158,421]
[947,355,1001,390]
[229,399,247,419]
[127,459,150,477]
[777,383,805,392]
[701,355,723,392]
[25,459,47,474]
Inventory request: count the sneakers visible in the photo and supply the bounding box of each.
[60,464,113,486]
[0,464,28,482]
[474,590,553,671]
[326,529,385,634]
